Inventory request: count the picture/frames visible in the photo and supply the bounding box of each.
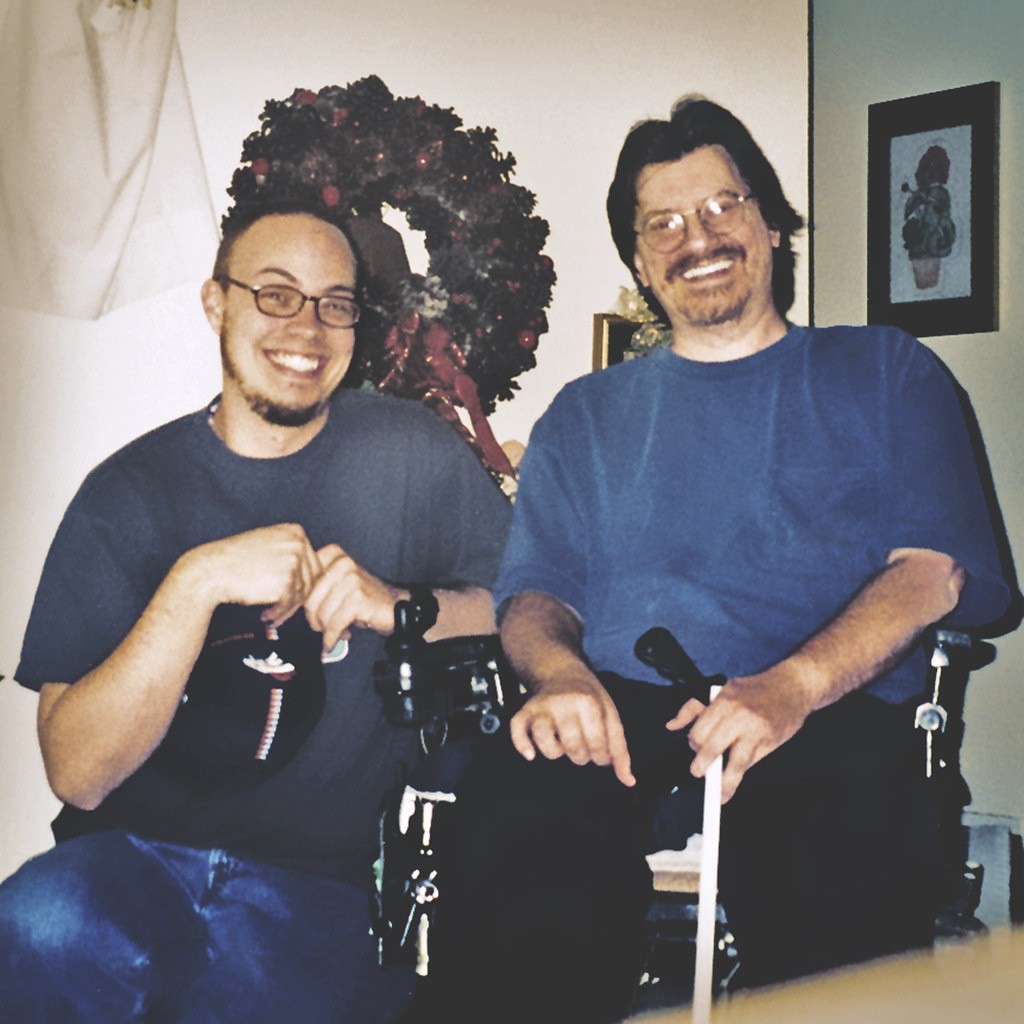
[865,78,1000,336]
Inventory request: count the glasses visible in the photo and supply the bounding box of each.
[632,190,756,253]
[213,274,362,329]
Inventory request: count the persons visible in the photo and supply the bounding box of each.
[387,93,1018,1024]
[0,195,516,1024]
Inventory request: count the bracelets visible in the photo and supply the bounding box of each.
[407,586,439,636]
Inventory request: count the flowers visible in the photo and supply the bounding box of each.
[216,74,558,418]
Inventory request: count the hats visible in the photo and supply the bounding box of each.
[173,604,324,791]
